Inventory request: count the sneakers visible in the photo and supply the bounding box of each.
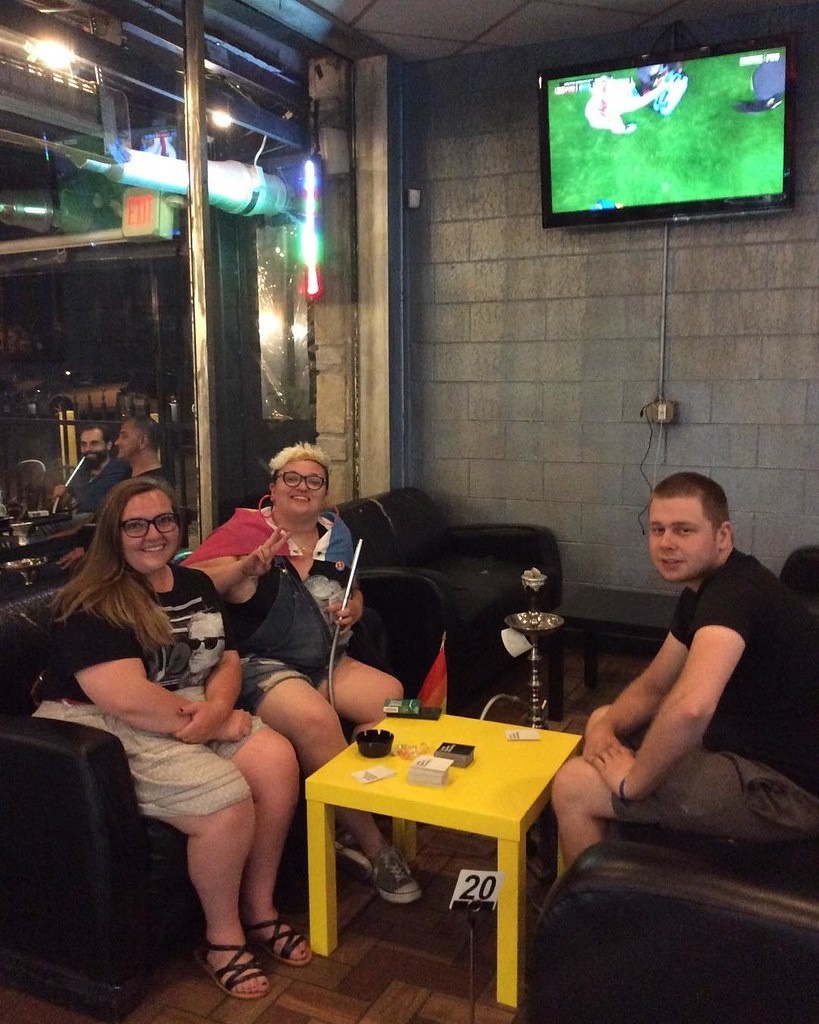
[336,821,373,871]
[367,846,421,904]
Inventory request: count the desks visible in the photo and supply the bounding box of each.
[305,713,584,1008]
[0,513,95,550]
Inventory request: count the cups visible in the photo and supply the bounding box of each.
[10,522,33,546]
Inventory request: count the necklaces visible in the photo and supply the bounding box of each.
[301,546,306,550]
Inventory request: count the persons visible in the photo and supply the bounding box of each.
[550,472,819,872]
[585,63,688,134]
[51,416,169,570]
[176,439,423,905]
[31,478,313,998]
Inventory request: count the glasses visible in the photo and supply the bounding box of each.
[274,472,326,490]
[122,512,180,537]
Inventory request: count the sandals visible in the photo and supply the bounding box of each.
[242,909,312,967]
[193,937,268,998]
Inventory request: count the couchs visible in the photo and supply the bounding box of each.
[0,549,447,1024]
[529,545,819,1024]
[332,487,562,712]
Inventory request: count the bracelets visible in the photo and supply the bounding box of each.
[619,776,627,801]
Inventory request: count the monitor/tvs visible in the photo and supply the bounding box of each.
[538,32,798,230]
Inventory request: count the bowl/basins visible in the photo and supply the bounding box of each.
[0,556,47,587]
